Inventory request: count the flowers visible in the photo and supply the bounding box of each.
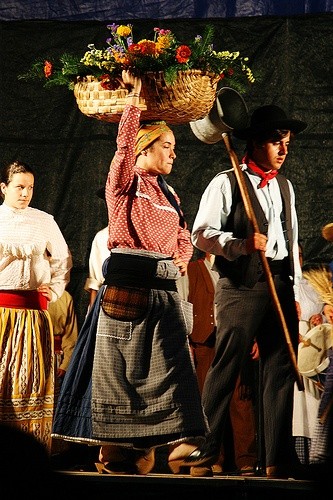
[17,23,255,96]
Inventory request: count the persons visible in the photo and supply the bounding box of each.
[48,226,333,478]
[191,107,303,479]
[51,70,219,477]
[0,160,73,449]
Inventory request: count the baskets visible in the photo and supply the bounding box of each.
[73,68,220,125]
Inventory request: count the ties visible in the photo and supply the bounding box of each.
[242,156,278,188]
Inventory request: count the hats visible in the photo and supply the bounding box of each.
[233,105,307,140]
[134,121,173,157]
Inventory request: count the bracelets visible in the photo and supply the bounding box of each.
[126,92,139,97]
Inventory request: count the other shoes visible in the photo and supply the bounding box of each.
[190,465,213,477]
[211,464,225,476]
[135,448,154,475]
[266,466,296,478]
[240,465,257,477]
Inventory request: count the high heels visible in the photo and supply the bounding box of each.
[94,457,139,474]
[168,447,218,473]
